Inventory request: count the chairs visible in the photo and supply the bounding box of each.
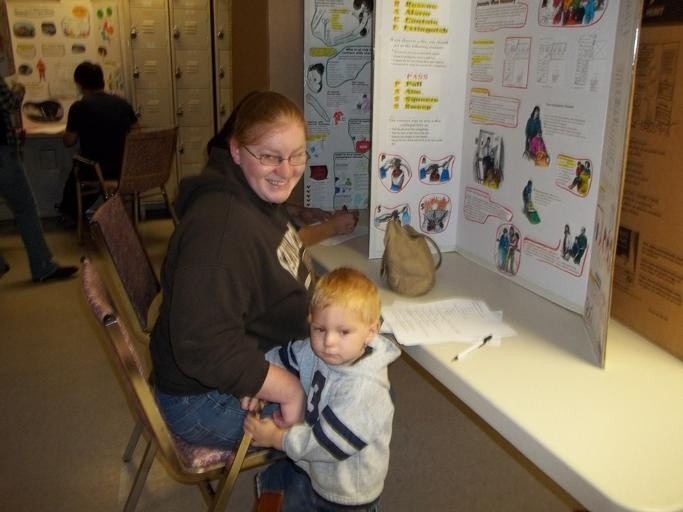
[77,190,288,512]
[71,125,180,233]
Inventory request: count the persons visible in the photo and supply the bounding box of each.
[55,61,141,230]
[282,200,359,248]
[561,225,572,257]
[496,226,520,275]
[523,179,533,215]
[151,87,317,511]
[240,267,402,512]
[569,161,592,196]
[0,0,80,284]
[523,104,548,163]
[570,226,589,265]
[481,135,494,182]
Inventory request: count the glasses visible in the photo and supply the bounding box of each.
[242,142,309,167]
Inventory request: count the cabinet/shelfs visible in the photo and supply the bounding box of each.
[116,1,235,223]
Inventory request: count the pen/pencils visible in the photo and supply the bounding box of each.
[343,204,348,214]
[452,335,490,363]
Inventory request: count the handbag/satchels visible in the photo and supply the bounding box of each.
[379,220,441,297]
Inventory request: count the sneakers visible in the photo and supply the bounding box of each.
[41,267,77,282]
